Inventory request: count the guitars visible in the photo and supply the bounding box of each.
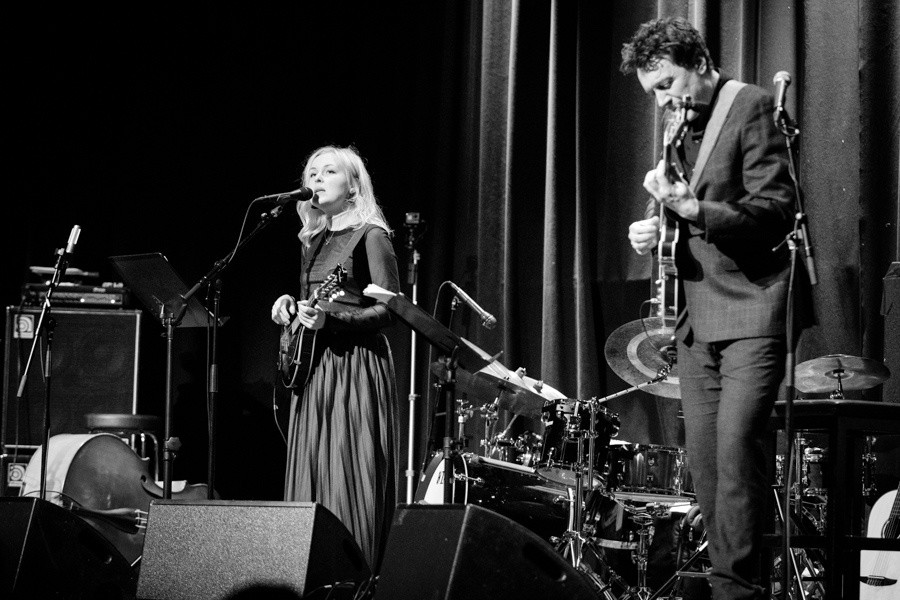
[866,484,900,539]
[278,265,348,389]
[653,94,698,329]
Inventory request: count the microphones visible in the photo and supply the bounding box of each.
[265,185,312,202]
[60,225,81,255]
[441,282,496,330]
[771,70,792,121]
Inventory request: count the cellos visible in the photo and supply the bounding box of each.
[20,432,224,543]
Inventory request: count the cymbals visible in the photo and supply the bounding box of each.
[793,352,891,394]
[519,373,569,403]
[430,360,547,420]
[603,314,680,401]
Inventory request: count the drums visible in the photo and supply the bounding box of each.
[606,440,699,505]
[413,449,555,543]
[535,397,622,490]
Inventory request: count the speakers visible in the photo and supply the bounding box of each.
[367,503,584,599]
[1,306,141,469]
[139,491,371,599]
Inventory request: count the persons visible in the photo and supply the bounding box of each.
[270,146,408,579]
[619,16,819,600]
